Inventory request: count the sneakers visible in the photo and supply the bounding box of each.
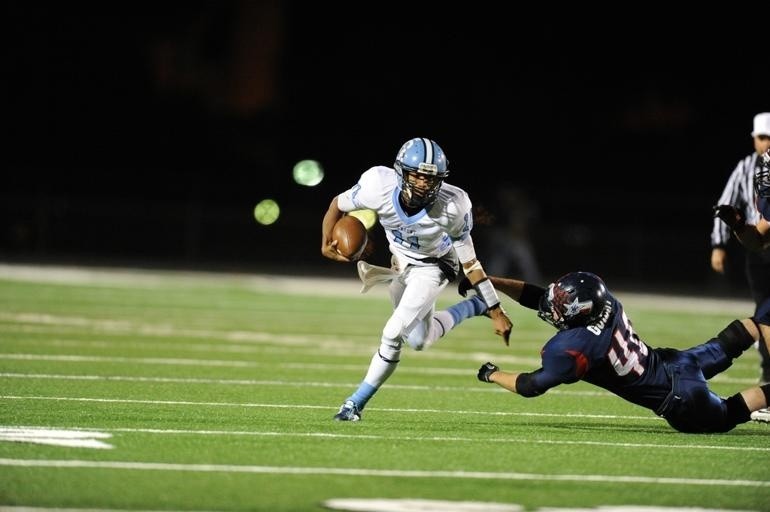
[750,409,770,422]
[334,400,361,421]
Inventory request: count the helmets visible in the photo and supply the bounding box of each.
[538,272,608,330]
[754,149,770,198]
[393,138,449,207]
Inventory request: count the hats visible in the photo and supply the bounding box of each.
[752,113,770,137]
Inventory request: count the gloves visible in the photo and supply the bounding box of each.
[458,278,472,297]
[717,205,746,229]
[478,362,498,383]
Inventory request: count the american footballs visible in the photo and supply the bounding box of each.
[332,216,368,261]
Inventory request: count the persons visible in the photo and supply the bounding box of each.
[321,139,513,421]
[710,112,770,274]
[712,189,768,422]
[458,270,769,434]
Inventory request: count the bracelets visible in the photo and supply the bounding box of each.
[472,277,500,310]
